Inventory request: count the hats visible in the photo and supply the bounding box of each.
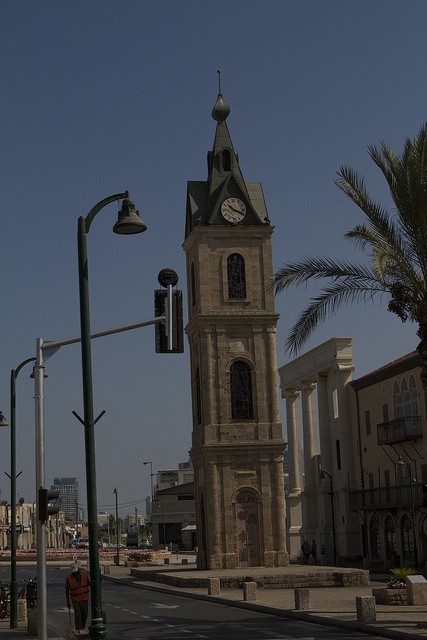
[71,563,79,574]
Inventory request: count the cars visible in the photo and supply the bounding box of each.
[73,538,89,549]
[139,539,150,547]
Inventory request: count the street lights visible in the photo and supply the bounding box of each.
[142,460,153,504]
[319,470,337,566]
[155,502,167,548]
[3,357,48,628]
[113,488,119,566]
[397,455,420,570]
[72,190,147,639]
[149,473,158,492]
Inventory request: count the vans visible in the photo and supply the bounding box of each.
[126,532,138,547]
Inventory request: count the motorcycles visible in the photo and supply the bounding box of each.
[24,576,38,608]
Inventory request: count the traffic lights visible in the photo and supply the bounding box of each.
[38,485,59,524]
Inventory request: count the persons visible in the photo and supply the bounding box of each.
[64,562,103,634]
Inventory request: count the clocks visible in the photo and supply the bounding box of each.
[220,196,247,224]
